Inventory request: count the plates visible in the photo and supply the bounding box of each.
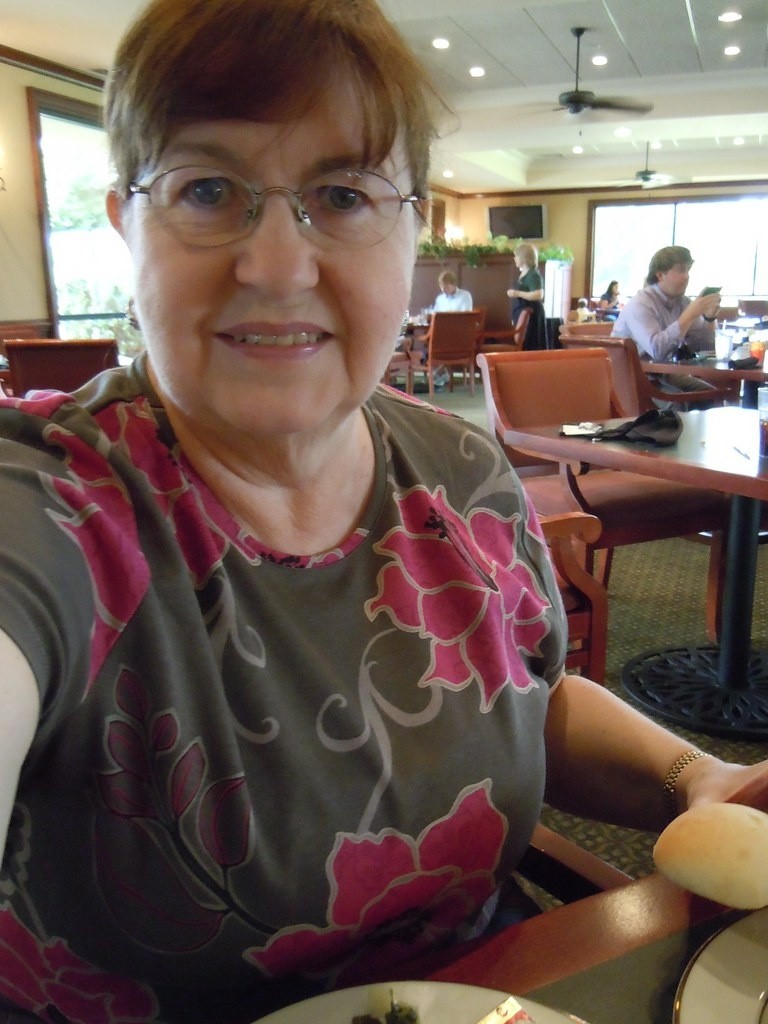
[672,906,768,1024]
[249,981,591,1024]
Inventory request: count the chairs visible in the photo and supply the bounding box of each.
[0,308,768,939]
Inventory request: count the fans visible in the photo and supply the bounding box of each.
[607,140,666,186]
[522,27,654,115]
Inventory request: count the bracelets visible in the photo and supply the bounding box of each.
[702,311,718,322]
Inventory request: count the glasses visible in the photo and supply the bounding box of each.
[128,164,423,251]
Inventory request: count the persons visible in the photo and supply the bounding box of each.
[1,0,768,1024]
[607,245,720,361]
[598,279,625,323]
[426,270,473,392]
[506,243,550,351]
[575,298,594,322]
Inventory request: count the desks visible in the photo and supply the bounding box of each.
[639,356,768,408]
[404,324,430,357]
[425,873,750,1024]
[502,402,768,744]
[596,309,621,314]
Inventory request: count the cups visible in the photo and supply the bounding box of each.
[757,387,768,459]
[749,341,766,368]
[714,329,734,363]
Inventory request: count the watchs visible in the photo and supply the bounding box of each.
[661,747,717,839]
